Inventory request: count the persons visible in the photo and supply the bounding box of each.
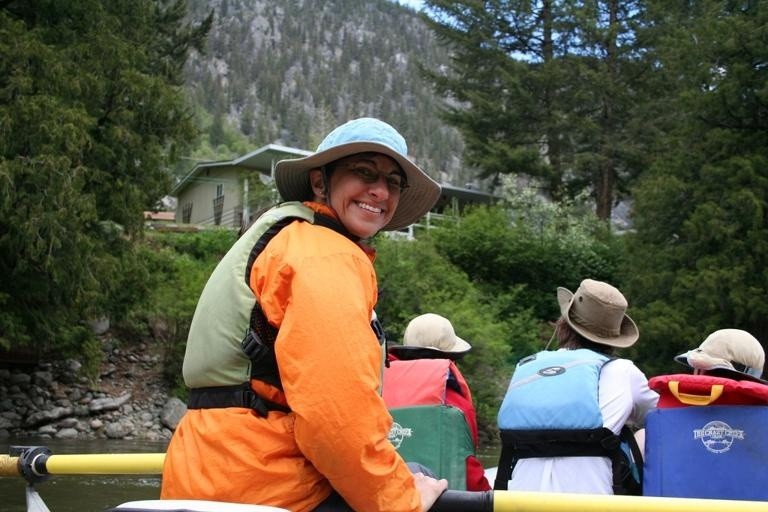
[494,278,659,494]
[159,115,447,511]
[649,327,767,409]
[385,314,491,491]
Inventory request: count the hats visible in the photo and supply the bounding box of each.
[389,312,474,360]
[674,327,765,382]
[554,278,641,349]
[274,117,443,234]
